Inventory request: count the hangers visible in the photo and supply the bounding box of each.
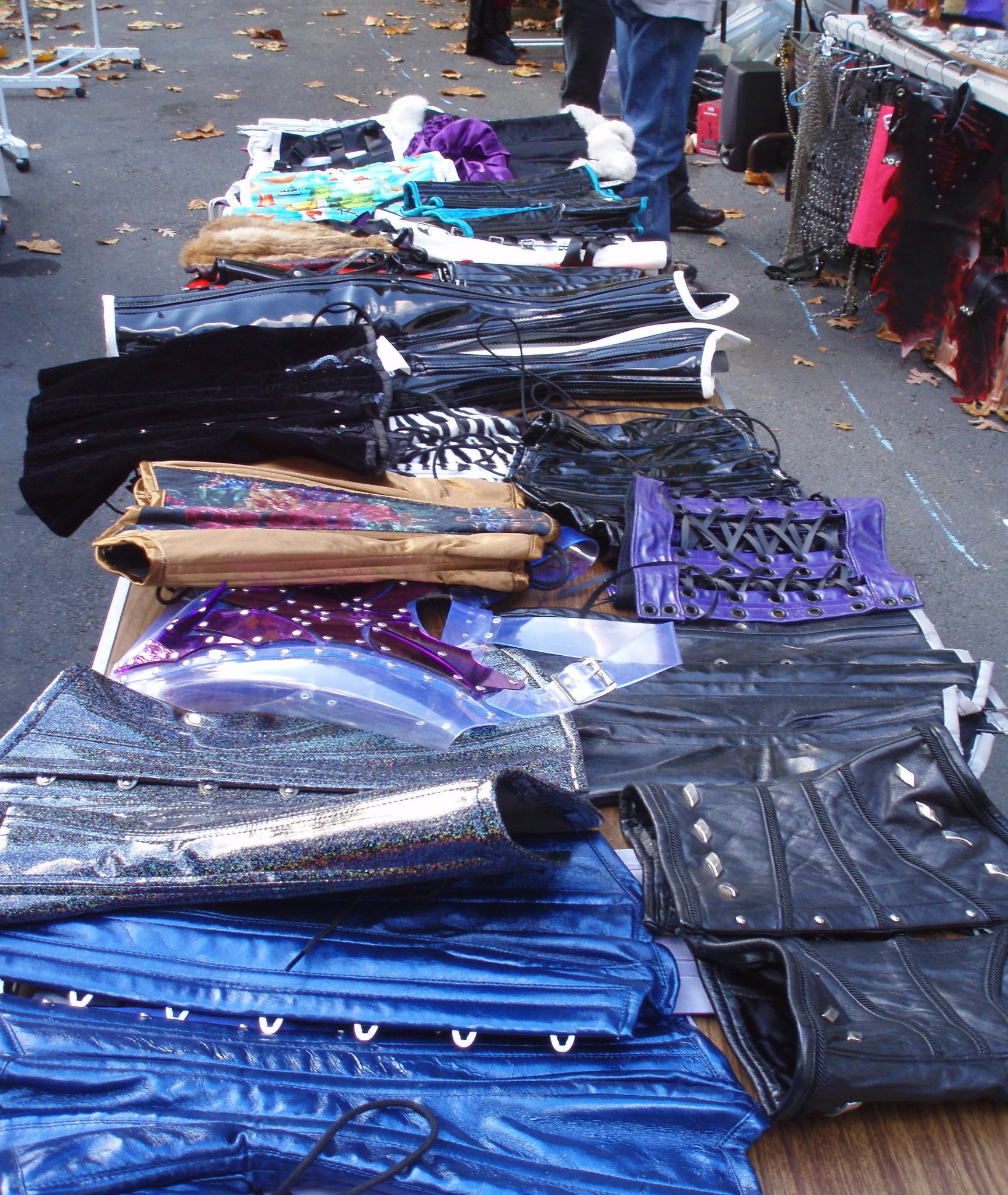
[789,10,996,156]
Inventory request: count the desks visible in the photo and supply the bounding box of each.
[92,119,1008,1195]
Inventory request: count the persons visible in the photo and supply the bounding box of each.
[613,0,725,233]
[560,0,616,116]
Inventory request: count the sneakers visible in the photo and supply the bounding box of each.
[669,202,725,231]
[658,258,697,284]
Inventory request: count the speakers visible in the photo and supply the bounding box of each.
[718,61,783,172]
